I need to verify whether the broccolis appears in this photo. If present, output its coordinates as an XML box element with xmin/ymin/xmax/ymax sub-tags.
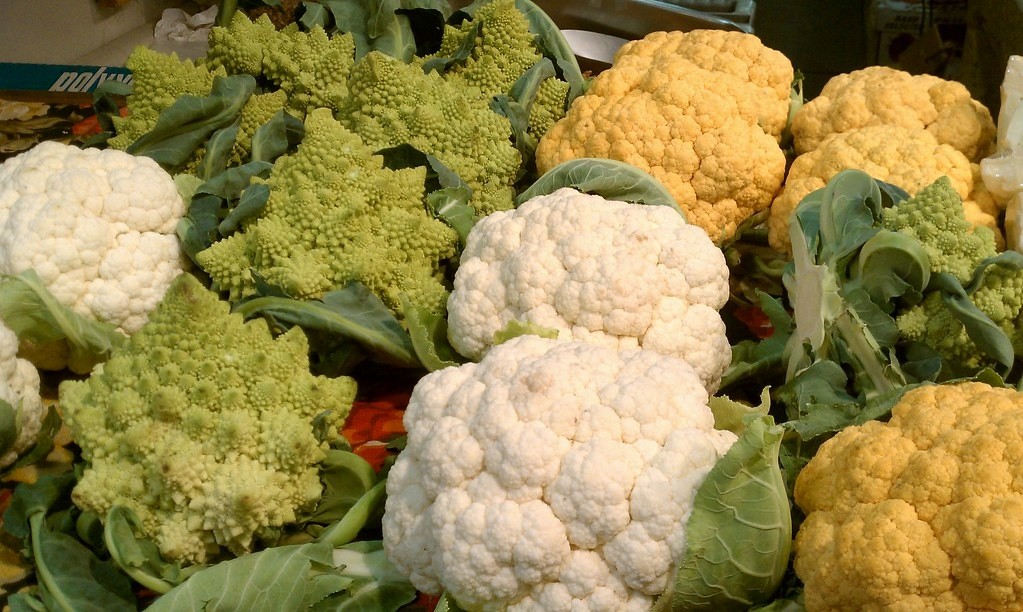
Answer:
<box><xmin>0</xmin><ymin>0</ymin><xmax>1023</xmax><ymax>612</ymax></box>
